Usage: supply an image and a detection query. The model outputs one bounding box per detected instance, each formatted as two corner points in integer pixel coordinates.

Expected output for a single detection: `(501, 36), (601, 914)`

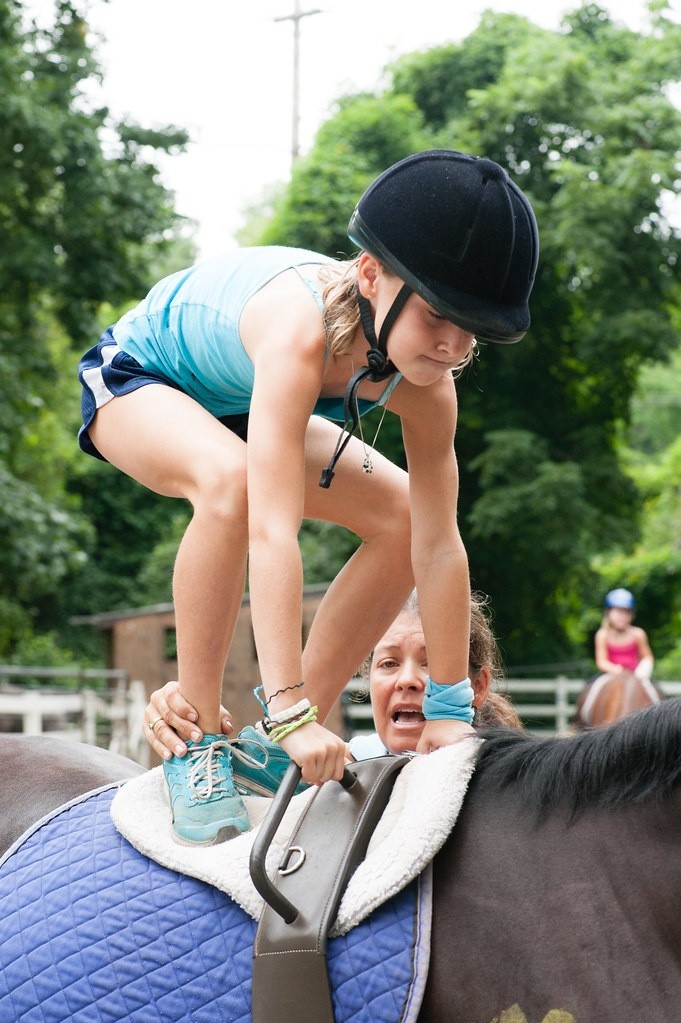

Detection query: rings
(148, 716), (163, 731)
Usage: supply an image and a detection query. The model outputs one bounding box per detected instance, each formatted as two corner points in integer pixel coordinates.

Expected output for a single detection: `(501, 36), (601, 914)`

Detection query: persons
(74, 149), (542, 849)
(141, 579), (531, 772)
(578, 588), (662, 725)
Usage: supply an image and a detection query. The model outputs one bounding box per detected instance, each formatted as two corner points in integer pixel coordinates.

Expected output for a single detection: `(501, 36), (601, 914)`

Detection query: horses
(0, 669), (681, 1023)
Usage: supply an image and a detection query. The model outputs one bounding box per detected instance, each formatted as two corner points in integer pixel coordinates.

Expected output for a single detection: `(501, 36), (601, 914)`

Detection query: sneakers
(231, 725), (316, 797)
(162, 734), (271, 848)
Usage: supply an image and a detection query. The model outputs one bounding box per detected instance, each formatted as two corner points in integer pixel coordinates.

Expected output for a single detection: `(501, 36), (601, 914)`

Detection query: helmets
(347, 148), (540, 344)
(604, 588), (635, 610)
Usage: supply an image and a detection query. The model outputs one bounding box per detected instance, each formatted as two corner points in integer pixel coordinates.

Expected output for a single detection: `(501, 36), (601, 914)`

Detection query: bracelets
(252, 679), (317, 743)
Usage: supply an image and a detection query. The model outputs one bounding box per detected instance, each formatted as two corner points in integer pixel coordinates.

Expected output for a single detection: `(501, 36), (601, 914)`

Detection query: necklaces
(350, 355), (396, 473)
